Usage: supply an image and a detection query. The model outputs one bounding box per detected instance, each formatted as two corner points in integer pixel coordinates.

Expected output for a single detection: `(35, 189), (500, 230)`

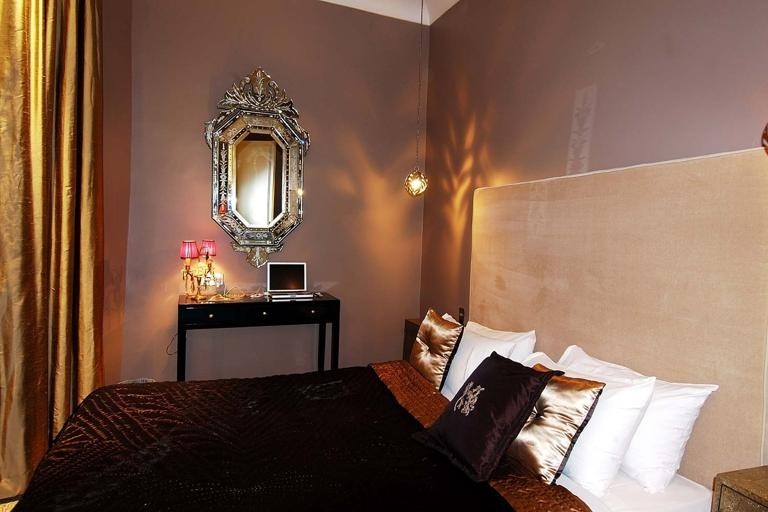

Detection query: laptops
(267, 262), (313, 299)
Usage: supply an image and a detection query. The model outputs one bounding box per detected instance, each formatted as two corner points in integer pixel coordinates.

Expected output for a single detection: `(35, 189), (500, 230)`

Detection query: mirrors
(204, 66), (310, 269)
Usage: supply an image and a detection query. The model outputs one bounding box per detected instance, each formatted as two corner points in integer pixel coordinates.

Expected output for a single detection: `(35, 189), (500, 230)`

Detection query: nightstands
(710, 465), (768, 511)
(404, 318), (423, 360)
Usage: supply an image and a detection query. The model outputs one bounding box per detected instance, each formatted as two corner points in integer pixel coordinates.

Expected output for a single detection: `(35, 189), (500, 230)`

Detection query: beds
(11, 146), (768, 512)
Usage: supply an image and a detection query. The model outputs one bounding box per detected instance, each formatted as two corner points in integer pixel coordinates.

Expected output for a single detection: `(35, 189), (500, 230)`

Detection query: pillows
(410, 309), (464, 393)
(557, 345), (719, 496)
(524, 353), (656, 499)
(508, 363), (606, 485)
(441, 312), (517, 402)
(466, 321), (537, 362)
(413, 351), (566, 486)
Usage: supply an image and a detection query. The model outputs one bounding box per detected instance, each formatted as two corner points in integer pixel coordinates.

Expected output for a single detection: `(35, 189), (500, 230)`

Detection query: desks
(178, 292), (340, 381)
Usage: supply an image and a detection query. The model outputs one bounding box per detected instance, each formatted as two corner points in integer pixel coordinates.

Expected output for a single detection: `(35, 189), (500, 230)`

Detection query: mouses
(315, 292), (324, 298)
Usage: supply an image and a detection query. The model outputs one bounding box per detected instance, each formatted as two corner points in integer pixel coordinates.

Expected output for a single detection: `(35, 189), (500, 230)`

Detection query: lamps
(179, 239), (217, 301)
(406, 0), (430, 198)
